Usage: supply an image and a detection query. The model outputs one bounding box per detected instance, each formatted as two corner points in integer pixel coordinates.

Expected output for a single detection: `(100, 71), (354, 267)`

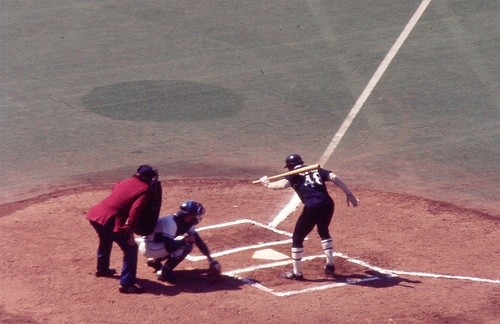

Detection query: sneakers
(323, 265), (335, 274)
(284, 272), (304, 280)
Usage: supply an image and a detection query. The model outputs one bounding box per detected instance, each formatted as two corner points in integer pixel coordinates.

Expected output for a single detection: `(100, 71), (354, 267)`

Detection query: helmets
(283, 154), (303, 169)
(137, 165), (158, 182)
(175, 200), (202, 218)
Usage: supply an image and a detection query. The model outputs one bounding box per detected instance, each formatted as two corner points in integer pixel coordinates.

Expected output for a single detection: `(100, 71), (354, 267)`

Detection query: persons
(259, 154), (360, 281)
(84, 164), (162, 293)
(140, 201), (220, 278)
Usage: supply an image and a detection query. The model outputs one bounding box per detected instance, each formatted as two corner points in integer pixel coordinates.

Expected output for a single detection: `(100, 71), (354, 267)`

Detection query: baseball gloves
(207, 259), (221, 283)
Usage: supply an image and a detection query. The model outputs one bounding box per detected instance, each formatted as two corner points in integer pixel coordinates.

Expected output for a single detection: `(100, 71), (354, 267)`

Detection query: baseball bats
(252, 163), (321, 184)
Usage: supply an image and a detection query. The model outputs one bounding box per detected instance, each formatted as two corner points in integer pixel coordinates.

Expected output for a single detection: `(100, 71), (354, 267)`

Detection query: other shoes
(157, 270), (181, 282)
(147, 259), (162, 272)
(96, 268), (116, 276)
(119, 283), (144, 293)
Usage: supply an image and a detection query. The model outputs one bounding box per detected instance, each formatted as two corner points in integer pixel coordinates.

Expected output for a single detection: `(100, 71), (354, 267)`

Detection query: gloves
(260, 176), (270, 187)
(347, 194), (358, 207)
